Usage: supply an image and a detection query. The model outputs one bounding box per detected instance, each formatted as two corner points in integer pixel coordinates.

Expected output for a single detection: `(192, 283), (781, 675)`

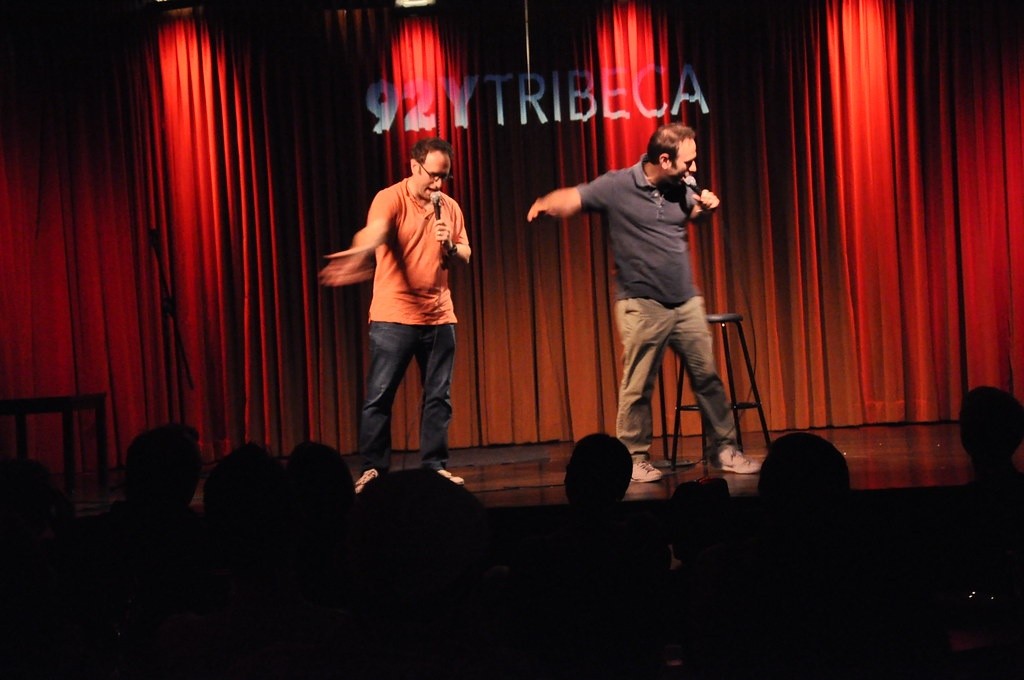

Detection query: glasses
(416, 159), (454, 185)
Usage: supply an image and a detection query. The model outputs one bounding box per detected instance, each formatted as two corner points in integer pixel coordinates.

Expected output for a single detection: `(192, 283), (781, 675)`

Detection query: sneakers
(629, 461), (664, 483)
(710, 444), (767, 474)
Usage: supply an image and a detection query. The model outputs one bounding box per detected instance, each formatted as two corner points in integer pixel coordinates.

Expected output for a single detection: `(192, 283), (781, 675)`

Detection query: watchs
(447, 245), (457, 255)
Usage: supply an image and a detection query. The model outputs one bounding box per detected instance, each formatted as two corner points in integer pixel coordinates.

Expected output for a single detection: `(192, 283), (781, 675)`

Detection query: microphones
(684, 175), (702, 196)
(430, 192), (442, 221)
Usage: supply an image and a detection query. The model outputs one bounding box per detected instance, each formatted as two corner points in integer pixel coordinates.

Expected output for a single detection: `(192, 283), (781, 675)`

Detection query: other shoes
(437, 469), (464, 485)
(355, 469), (378, 493)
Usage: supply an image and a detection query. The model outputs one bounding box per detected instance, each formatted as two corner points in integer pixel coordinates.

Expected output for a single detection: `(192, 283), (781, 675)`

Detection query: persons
(527, 124), (762, 482)
(320, 138), (472, 491)
(0, 385), (1024, 680)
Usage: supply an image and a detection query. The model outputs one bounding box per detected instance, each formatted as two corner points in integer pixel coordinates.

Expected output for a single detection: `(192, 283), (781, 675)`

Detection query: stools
(668, 314), (771, 469)
